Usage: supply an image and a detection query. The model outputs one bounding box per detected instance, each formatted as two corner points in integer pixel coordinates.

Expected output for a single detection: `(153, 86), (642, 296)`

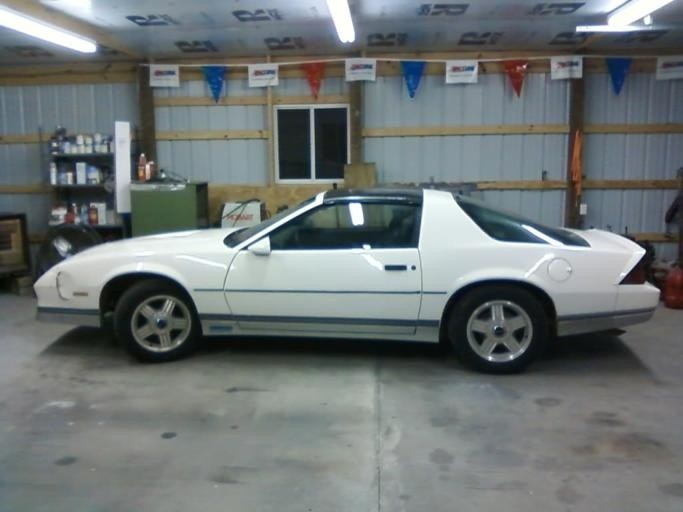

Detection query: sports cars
(32, 185), (661, 374)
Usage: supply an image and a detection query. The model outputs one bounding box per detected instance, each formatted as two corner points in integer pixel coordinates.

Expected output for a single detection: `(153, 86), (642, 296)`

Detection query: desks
(130, 180), (210, 239)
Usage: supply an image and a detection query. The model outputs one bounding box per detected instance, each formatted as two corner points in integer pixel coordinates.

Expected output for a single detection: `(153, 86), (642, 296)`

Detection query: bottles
(138, 153), (147, 179)
(48, 160), (74, 185)
(66, 202), (99, 225)
(50, 125), (110, 154)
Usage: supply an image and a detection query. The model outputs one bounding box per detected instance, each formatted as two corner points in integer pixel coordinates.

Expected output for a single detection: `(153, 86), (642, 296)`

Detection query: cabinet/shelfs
(48, 136), (130, 264)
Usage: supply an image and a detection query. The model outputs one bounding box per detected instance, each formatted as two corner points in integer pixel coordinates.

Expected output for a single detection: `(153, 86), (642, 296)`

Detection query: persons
(665, 167), (683, 268)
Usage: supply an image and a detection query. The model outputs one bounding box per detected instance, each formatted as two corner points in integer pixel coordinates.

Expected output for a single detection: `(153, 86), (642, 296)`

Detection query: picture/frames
(1, 212), (34, 280)
